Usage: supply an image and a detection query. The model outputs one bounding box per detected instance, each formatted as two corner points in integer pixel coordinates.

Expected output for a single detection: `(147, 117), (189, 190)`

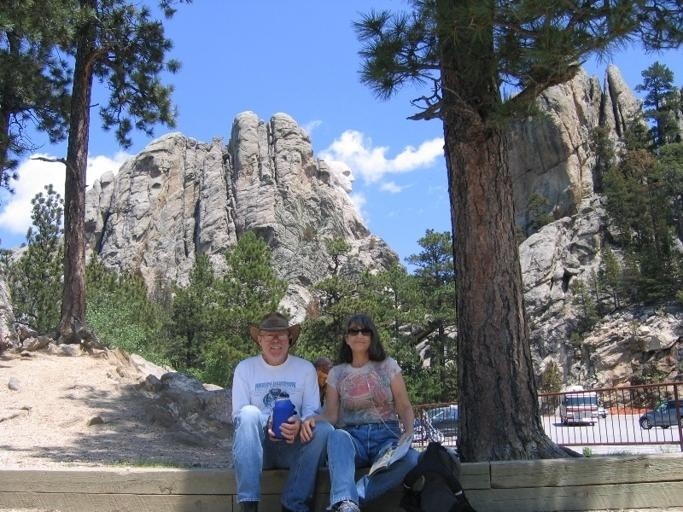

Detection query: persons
(299, 313), (422, 512)
(229, 311), (336, 512)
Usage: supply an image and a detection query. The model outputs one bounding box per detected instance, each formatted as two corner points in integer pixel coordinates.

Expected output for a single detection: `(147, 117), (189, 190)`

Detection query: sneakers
(338, 499), (362, 511)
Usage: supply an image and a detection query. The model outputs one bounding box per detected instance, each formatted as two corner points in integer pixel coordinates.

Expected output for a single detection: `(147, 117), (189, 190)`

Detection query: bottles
(272, 392), (294, 440)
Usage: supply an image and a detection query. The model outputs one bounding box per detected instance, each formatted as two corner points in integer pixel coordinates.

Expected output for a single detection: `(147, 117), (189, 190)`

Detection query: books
(368, 434), (413, 477)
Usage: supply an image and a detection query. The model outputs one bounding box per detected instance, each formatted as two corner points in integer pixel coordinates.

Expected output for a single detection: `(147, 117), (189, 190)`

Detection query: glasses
(257, 331), (289, 342)
(347, 327), (373, 337)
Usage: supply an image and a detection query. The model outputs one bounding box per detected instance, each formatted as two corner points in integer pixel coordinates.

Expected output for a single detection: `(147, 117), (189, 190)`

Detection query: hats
(247, 312), (301, 348)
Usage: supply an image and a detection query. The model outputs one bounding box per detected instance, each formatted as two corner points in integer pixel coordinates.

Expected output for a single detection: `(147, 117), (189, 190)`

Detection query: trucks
(556, 385), (598, 427)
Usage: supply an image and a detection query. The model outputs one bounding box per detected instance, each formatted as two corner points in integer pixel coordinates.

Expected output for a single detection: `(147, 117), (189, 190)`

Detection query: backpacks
(402, 442), (472, 512)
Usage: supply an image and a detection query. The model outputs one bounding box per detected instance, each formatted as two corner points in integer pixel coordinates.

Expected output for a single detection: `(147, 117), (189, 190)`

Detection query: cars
(638, 399), (683, 430)
(597, 406), (608, 419)
(414, 404), (458, 435)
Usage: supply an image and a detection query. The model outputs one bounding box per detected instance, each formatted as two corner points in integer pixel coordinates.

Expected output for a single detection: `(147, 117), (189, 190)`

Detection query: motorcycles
(398, 409), (444, 445)
